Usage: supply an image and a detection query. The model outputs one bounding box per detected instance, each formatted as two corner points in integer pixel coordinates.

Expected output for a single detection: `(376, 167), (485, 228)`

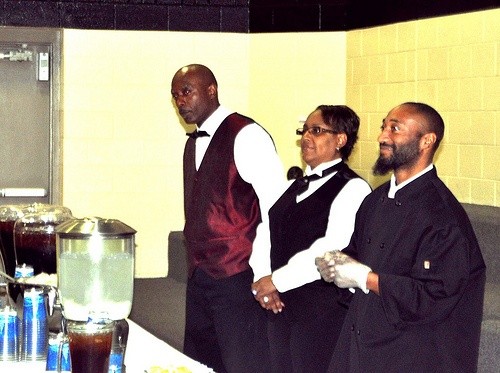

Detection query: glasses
(296, 127), (337, 137)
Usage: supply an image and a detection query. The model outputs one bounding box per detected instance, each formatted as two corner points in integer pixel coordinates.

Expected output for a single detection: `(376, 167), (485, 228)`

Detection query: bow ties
(186, 130), (210, 139)
(286, 166), (310, 195)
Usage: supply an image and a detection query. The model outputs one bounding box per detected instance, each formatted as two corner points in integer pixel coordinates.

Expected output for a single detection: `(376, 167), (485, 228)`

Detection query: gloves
(330, 249), (372, 295)
(314, 250), (335, 283)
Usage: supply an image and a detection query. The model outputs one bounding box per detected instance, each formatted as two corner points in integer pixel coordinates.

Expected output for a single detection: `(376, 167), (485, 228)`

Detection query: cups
(0, 267), (124, 373)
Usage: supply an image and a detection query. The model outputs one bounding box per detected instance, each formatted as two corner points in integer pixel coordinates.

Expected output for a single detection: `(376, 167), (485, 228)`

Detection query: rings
(263, 296), (269, 302)
(252, 289), (257, 296)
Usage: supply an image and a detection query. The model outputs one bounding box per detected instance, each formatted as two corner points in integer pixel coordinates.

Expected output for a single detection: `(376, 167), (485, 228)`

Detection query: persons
(171, 64), (291, 373)
(315, 101), (486, 373)
(249, 105), (373, 373)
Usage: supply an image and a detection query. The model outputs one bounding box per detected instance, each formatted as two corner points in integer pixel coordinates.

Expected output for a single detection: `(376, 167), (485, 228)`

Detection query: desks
(0, 318), (216, 373)
